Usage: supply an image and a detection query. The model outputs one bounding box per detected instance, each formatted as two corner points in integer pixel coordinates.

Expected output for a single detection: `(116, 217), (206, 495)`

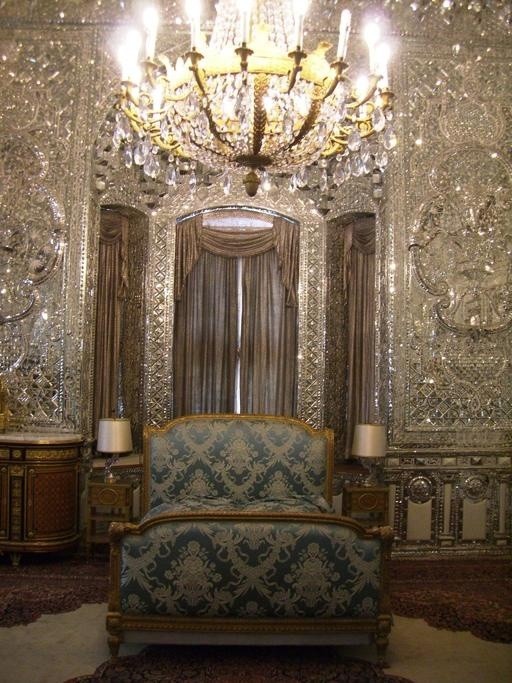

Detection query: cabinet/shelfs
(0, 432), (85, 567)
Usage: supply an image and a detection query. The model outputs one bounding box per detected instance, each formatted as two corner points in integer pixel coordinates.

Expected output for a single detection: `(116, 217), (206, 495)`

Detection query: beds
(108, 414), (394, 669)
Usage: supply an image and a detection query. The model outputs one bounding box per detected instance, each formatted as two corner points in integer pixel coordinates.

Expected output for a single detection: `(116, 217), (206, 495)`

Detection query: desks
(343, 483), (390, 560)
(86, 477), (142, 563)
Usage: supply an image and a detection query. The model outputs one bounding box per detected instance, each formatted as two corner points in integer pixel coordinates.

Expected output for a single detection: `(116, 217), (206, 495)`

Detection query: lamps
(97, 418), (133, 483)
(112, 0), (398, 196)
(351, 422), (387, 488)
